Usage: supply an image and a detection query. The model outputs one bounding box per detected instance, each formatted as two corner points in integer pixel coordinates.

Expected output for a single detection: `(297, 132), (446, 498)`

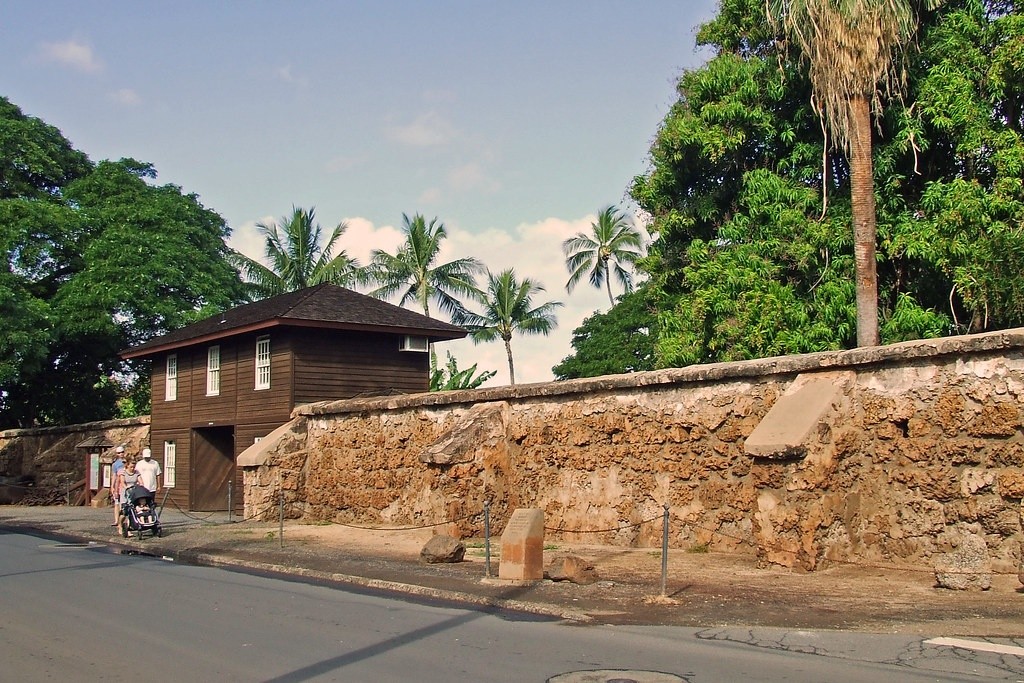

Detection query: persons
(109, 446), (129, 527)
(117, 459), (144, 537)
(135, 497), (155, 526)
(134, 448), (162, 507)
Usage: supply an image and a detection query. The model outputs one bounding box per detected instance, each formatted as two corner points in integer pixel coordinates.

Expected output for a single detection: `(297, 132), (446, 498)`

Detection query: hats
(143, 449), (152, 457)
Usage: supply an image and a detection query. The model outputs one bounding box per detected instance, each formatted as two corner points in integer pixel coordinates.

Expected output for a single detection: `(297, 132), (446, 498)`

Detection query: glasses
(117, 451), (124, 454)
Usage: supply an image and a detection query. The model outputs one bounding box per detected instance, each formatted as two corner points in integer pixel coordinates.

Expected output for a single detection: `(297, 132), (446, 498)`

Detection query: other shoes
(111, 522), (118, 526)
(128, 534), (133, 536)
(119, 528), (122, 535)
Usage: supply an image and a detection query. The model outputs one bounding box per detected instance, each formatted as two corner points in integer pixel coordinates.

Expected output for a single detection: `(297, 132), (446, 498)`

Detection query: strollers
(120, 485), (163, 540)
